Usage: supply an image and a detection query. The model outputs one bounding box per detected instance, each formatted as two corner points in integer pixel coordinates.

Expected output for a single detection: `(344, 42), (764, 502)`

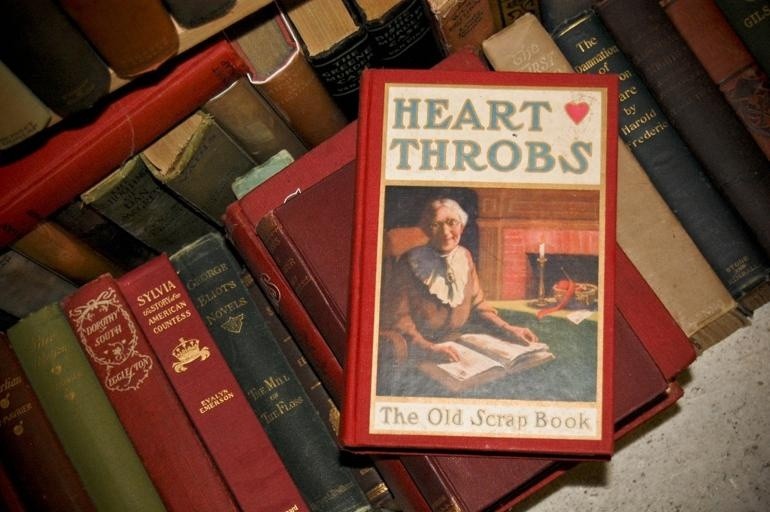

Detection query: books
(0, 0), (769, 512)
